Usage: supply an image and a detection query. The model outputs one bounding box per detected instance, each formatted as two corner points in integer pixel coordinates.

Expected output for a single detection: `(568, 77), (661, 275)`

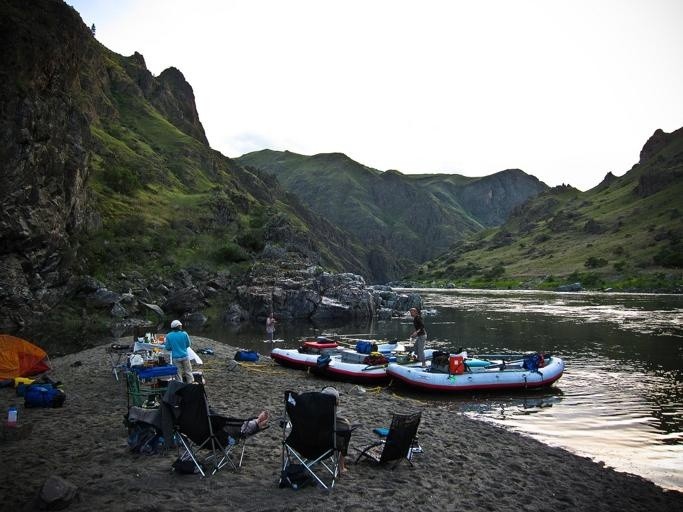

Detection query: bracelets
(416, 330), (417, 333)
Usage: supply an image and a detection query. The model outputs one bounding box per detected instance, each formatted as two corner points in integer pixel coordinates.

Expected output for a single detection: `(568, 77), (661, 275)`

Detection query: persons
(409, 307), (426, 366)
(163, 320), (194, 384)
(265, 312), (275, 340)
(321, 386), (350, 472)
(209, 406), (270, 446)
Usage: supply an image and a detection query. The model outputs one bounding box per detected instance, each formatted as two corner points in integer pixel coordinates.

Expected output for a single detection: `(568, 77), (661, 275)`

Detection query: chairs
(124, 370), (422, 492)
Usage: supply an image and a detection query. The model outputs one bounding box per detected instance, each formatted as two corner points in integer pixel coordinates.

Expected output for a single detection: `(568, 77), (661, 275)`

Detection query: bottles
(7, 407), (17, 428)
(142, 350), (165, 369)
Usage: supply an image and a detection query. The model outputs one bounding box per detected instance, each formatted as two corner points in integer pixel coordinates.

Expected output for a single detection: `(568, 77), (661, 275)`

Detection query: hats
(170, 319), (182, 329)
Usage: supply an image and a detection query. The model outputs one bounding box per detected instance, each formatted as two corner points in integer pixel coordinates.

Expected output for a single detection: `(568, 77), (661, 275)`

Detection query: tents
(0, 334), (53, 390)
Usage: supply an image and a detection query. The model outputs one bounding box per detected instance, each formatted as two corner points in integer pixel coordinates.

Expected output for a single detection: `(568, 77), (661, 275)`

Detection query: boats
(270, 336), (564, 398)
